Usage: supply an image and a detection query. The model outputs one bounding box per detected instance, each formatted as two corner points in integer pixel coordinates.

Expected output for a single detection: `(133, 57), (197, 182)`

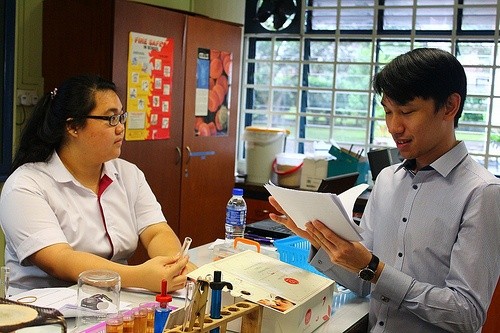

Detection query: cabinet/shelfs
(233, 179), (369, 226)
(42, 0), (247, 266)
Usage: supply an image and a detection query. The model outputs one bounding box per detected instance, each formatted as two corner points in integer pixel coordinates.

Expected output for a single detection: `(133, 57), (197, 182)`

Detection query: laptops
(244, 172), (360, 239)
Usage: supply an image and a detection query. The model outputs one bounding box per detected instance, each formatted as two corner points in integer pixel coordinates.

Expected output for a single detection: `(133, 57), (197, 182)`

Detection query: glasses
(87, 110), (129, 128)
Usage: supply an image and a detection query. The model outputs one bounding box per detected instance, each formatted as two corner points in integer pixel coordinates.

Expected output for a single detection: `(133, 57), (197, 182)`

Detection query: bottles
(224, 188), (247, 249)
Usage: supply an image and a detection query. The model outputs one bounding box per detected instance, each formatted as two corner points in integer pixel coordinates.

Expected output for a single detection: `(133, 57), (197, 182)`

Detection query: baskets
(271, 236), (353, 296)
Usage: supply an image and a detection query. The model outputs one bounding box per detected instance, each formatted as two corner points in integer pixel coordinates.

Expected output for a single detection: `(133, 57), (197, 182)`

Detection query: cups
(73, 270), (121, 333)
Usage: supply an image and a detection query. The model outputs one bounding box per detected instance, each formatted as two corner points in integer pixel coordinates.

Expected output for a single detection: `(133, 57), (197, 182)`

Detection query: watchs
(357, 254), (380, 282)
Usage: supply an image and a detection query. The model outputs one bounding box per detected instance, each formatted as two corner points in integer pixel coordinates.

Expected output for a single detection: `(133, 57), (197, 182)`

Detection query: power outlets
(17, 89), (38, 109)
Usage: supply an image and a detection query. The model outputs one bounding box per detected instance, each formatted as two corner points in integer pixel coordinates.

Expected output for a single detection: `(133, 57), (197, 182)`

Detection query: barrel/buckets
(272, 153), (306, 188)
(241, 125), (291, 186)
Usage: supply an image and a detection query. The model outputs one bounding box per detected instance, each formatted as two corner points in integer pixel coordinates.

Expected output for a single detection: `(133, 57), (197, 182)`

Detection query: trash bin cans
(243, 126), (291, 184)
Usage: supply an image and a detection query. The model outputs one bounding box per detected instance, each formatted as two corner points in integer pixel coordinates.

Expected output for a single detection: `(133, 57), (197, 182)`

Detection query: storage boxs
(300, 157), (356, 192)
(187, 250), (336, 332)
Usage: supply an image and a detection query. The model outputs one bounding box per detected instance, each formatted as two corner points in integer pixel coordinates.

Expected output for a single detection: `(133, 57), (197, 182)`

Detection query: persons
(259, 295), (295, 313)
(0, 76), (197, 299)
(268, 48), (500, 333)
